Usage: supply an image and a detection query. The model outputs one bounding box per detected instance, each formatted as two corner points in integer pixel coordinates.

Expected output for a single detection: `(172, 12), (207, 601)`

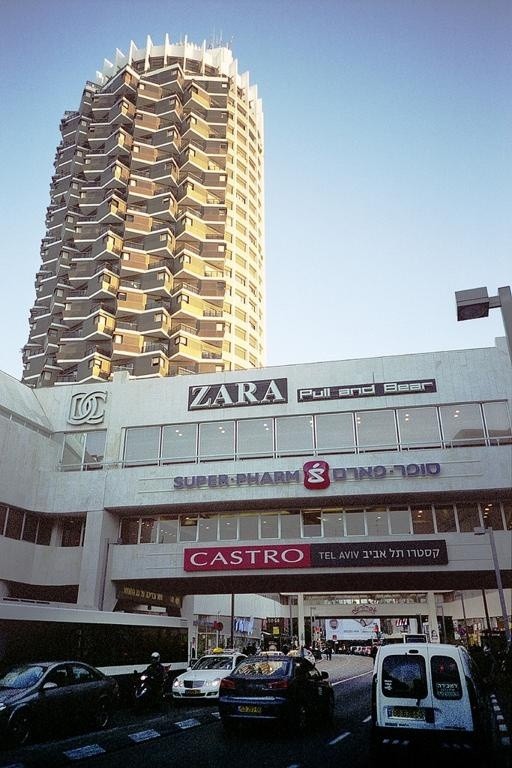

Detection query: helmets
(150, 652), (160, 662)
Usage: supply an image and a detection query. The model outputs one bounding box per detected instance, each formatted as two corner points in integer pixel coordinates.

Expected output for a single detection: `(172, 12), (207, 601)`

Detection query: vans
(369, 633), (487, 731)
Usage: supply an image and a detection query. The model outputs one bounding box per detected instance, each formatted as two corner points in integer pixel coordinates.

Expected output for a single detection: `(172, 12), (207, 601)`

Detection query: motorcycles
(128, 664), (173, 712)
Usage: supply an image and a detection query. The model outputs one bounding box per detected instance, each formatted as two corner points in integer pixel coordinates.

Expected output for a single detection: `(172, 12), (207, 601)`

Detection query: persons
(139, 650), (166, 697)
(326, 645), (333, 661)
(217, 636), (298, 656)
(371, 644), (378, 666)
(467, 640), (492, 670)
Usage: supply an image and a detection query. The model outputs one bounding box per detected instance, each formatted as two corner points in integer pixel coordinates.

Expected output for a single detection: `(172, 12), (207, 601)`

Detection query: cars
(349, 646), (378, 656)
(0, 661), (120, 745)
(172, 647), (335, 727)
